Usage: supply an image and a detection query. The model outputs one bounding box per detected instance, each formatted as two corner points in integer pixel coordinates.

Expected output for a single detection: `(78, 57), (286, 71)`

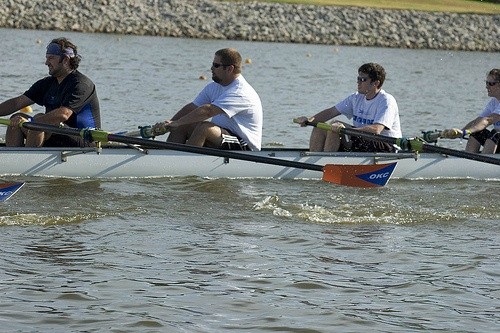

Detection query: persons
(152, 48), (263, 152)
(461, 68), (500, 154)
(297, 63), (403, 152)
(0, 37), (101, 148)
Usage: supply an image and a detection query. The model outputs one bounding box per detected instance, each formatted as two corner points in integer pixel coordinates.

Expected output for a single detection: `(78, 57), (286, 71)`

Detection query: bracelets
(463, 129), (471, 136)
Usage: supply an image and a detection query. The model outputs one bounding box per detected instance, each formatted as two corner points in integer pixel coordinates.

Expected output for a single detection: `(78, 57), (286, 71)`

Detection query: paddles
(423, 130), (471, 143)
(115, 122), (169, 137)
(293, 118), (500, 166)
(1, 178), (25, 205)
(1, 119), (399, 187)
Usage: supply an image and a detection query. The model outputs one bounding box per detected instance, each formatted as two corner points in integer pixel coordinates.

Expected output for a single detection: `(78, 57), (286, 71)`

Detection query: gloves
(443, 128), (461, 139)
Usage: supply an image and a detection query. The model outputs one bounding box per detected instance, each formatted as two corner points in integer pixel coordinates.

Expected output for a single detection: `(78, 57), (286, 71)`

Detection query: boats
(0, 106), (500, 180)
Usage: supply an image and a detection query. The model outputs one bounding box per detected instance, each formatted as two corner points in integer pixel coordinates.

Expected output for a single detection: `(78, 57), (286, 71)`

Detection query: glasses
(212, 62), (228, 68)
(486, 81), (500, 86)
(357, 76), (372, 82)
